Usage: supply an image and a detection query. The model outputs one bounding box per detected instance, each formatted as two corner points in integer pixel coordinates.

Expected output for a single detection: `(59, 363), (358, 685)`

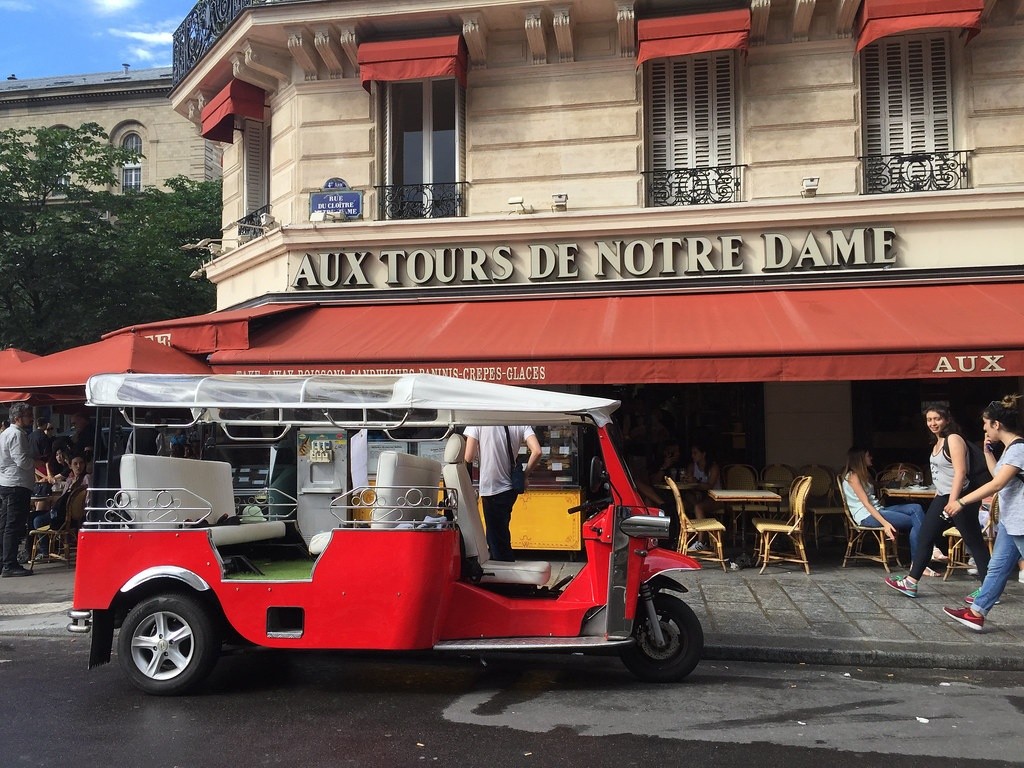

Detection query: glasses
(24, 414), (33, 417)
(46, 427), (54, 431)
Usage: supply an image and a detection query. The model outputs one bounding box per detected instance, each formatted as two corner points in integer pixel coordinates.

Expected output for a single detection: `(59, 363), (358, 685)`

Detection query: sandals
(931, 548), (950, 563)
(910, 564), (941, 577)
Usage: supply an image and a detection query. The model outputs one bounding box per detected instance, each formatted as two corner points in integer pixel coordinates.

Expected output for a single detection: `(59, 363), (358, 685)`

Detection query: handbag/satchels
(50, 489), (72, 530)
(511, 466), (525, 494)
(688, 490), (707, 505)
(943, 433), (994, 487)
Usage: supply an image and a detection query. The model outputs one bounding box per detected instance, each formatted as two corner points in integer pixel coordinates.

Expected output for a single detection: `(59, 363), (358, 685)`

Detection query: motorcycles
(68, 368), (706, 695)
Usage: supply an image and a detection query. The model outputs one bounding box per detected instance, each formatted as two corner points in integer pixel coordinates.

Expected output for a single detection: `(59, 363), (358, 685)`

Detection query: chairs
(27, 482), (89, 573)
(443, 433), (553, 586)
(661, 460), (1000, 582)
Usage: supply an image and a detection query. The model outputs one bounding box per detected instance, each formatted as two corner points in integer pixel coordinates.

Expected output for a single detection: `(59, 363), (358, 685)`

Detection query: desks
(707, 488), (782, 570)
(879, 484), (938, 566)
(650, 481), (700, 549)
(28, 495), (63, 563)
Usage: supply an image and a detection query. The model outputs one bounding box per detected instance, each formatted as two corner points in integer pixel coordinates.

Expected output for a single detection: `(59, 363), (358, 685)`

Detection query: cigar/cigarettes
(886, 538), (894, 540)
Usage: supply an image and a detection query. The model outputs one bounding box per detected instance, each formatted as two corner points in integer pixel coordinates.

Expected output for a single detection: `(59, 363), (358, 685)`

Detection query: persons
(942, 392), (1024, 632)
(886, 403), (1001, 603)
(463, 426), (540, 562)
(842, 444), (1024, 583)
(0, 401), (194, 577)
(624, 444), (721, 551)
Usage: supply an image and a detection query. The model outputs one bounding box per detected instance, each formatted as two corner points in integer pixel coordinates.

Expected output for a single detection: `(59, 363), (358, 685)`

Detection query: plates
(908, 486), (929, 490)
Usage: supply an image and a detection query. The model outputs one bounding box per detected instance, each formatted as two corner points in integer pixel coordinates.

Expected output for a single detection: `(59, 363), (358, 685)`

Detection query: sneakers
(687, 541), (711, 551)
(964, 586), (1000, 604)
(884, 576), (918, 597)
(943, 607), (984, 631)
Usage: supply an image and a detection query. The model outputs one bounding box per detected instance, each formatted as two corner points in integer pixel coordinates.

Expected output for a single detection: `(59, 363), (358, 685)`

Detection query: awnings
(851, 0), (985, 52)
(200, 79), (265, 143)
(635, 6), (752, 72)
(100, 278), (1024, 384)
(357, 33), (468, 94)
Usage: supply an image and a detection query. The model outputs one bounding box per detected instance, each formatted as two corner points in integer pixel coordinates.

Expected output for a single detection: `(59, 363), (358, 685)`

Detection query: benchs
(118, 453), (285, 546)
(307, 450), (441, 554)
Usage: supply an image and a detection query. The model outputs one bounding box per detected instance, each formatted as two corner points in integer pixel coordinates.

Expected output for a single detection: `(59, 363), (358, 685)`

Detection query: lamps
(180, 213), (281, 280)
(310, 211), (344, 222)
(508, 197), (535, 215)
(800, 177), (820, 198)
(551, 193), (569, 212)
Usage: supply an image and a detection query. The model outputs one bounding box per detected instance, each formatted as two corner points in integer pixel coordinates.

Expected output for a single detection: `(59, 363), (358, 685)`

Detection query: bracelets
(958, 499), (966, 507)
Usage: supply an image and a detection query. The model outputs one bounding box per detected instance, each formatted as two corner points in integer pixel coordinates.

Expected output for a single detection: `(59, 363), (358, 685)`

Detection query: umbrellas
(0, 328), (217, 509)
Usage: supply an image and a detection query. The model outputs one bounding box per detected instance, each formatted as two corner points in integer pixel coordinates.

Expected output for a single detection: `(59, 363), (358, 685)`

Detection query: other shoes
(1019, 570), (1024, 584)
(0, 562), (32, 576)
(31, 553), (48, 563)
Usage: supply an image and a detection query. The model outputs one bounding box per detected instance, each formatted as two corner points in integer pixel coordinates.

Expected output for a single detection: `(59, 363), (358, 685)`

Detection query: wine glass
(913, 471), (923, 489)
